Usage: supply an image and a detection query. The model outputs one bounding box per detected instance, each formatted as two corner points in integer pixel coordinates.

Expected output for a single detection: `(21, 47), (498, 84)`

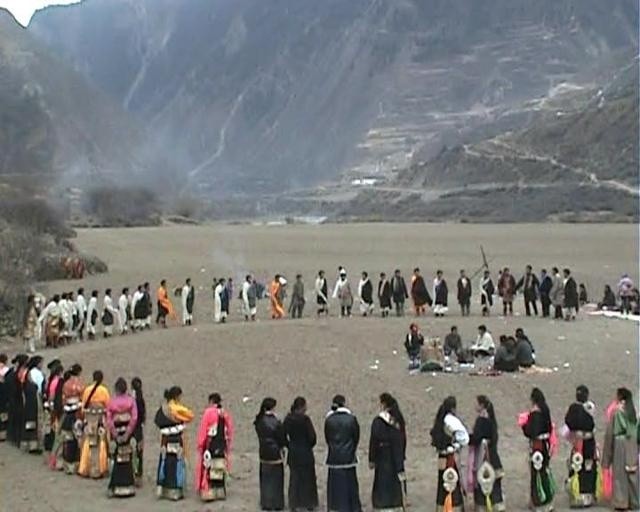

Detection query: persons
(0, 353), (148, 498)
(153, 386), (195, 501)
(23, 263), (579, 353)
(195, 394), (233, 501)
(577, 274), (640, 316)
(430, 394), (506, 512)
(404, 323), (535, 376)
(254, 392), (407, 512)
(518, 385), (640, 512)
